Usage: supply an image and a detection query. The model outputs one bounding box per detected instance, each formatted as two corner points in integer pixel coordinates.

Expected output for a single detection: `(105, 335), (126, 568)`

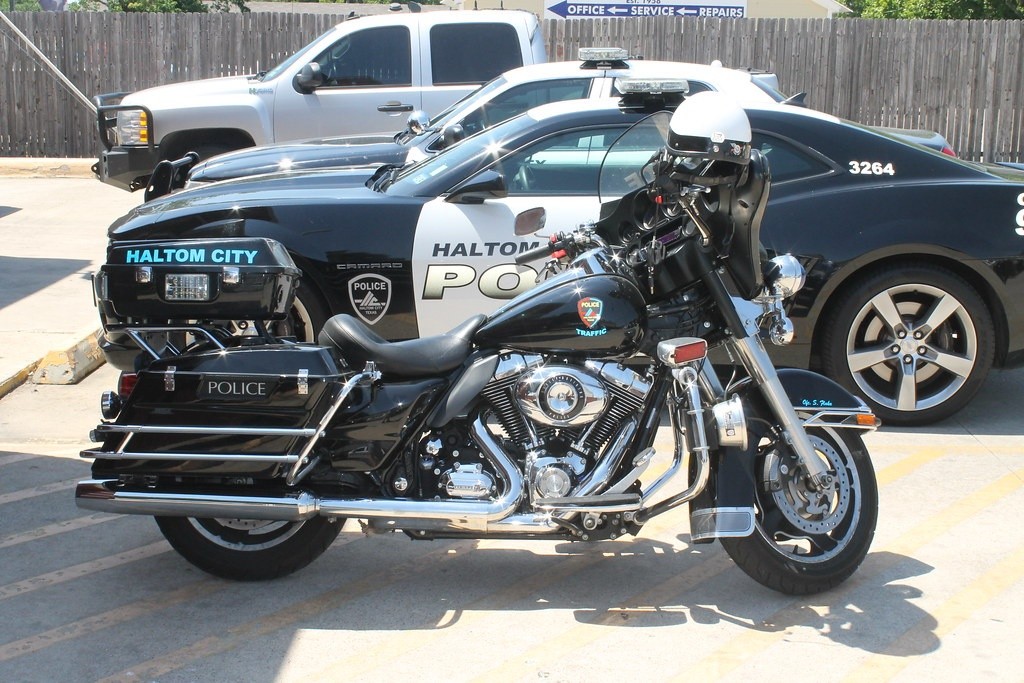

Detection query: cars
(91, 76), (1024, 428)
(141, 46), (962, 206)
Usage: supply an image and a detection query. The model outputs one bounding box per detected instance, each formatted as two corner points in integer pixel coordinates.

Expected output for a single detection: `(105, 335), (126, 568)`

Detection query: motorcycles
(75, 101), (883, 599)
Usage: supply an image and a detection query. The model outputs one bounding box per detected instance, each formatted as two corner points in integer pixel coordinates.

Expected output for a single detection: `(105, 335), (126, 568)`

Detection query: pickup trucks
(89, 9), (781, 193)
